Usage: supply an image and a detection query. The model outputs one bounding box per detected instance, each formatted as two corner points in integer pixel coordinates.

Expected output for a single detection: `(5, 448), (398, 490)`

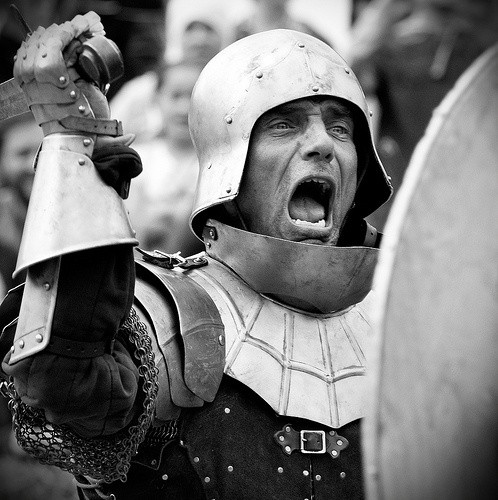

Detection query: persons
(108, 18), (226, 123)
(1, 118), (48, 295)
(231, 0), (332, 43)
(121, 61), (201, 258)
(0, 8), (394, 500)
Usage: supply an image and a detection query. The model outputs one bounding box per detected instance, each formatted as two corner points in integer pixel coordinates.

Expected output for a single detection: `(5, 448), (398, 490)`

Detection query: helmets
(188, 29), (392, 244)
